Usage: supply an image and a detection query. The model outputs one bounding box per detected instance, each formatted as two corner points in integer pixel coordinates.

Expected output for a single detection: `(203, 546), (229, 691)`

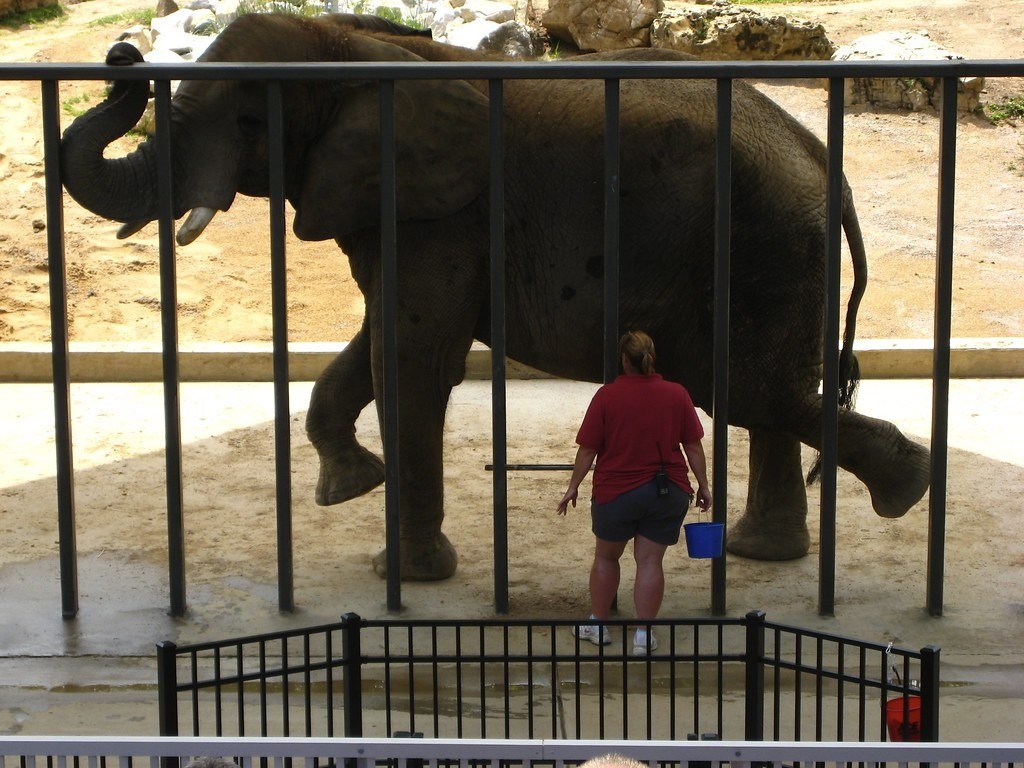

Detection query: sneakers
(572, 615), (612, 645)
(634, 627), (658, 656)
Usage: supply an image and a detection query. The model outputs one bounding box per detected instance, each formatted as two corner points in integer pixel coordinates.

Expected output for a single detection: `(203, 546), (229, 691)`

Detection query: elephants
(57, 11), (928, 583)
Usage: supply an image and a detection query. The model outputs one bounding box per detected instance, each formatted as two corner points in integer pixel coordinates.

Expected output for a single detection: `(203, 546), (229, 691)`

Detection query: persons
(557, 332), (713, 657)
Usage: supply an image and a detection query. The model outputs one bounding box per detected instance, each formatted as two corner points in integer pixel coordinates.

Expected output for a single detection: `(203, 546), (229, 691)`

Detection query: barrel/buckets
(683, 522), (725, 559)
(887, 696), (921, 742)
